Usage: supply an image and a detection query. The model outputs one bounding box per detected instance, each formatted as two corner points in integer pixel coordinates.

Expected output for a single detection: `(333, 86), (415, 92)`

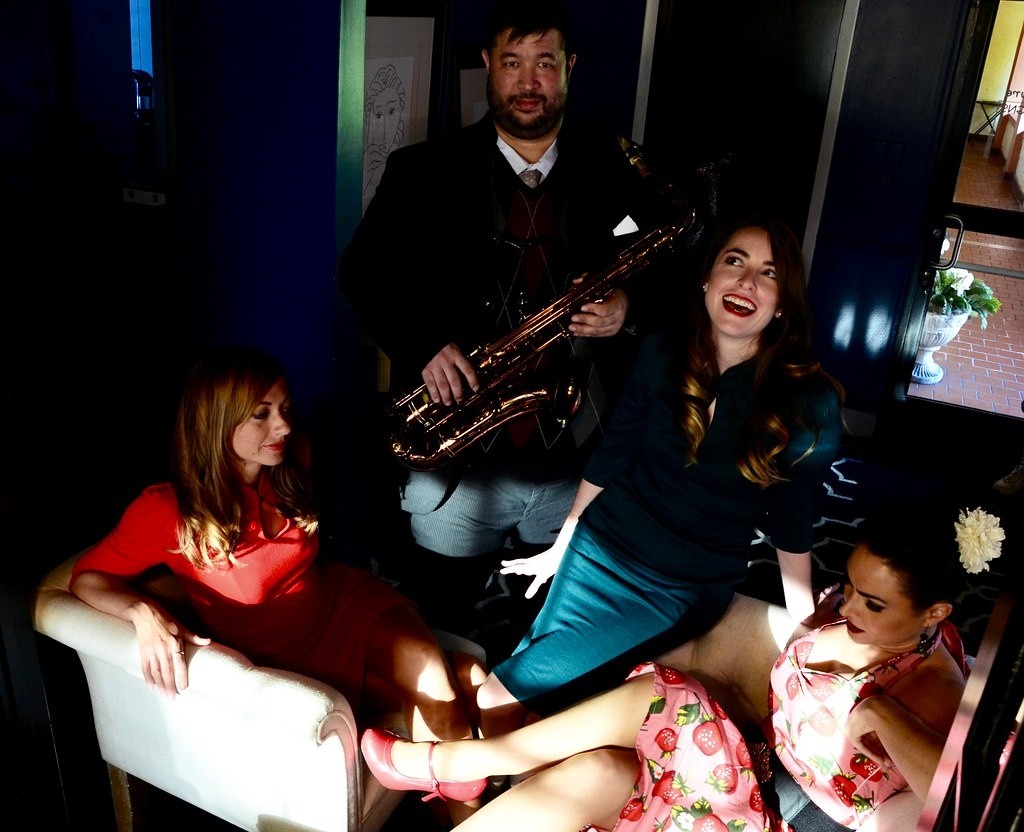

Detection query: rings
(174, 649), (184, 655)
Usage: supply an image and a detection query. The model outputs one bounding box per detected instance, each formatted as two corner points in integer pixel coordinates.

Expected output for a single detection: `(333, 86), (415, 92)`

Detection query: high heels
(360, 728), (489, 802)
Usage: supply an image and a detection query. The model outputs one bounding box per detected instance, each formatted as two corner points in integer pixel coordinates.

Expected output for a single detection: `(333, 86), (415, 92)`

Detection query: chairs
(32, 538), (486, 832)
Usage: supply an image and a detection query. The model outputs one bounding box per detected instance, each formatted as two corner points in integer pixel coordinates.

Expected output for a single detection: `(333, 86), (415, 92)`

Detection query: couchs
(648, 593), (924, 832)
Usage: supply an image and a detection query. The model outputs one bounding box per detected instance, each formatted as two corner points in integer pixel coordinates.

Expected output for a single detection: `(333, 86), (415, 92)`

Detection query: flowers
(953, 506), (1006, 575)
(928, 239), (1002, 330)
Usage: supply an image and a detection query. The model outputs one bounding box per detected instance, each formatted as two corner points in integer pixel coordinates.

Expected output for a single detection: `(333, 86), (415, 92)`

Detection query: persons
(69, 7), (968, 832)
(361, 490), (1005, 832)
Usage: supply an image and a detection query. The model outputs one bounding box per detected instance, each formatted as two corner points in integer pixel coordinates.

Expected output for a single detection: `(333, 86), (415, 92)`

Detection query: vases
(912, 306), (970, 384)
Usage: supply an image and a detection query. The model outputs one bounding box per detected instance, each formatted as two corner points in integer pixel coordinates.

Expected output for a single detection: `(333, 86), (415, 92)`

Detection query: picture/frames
(361, 0), (450, 219)
(452, 59), (490, 129)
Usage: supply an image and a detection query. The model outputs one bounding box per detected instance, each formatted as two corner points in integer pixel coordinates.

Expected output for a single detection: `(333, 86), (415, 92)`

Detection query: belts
(747, 727), (852, 832)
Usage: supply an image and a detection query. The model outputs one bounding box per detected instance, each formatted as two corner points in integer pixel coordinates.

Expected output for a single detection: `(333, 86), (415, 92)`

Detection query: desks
(968, 100), (1003, 143)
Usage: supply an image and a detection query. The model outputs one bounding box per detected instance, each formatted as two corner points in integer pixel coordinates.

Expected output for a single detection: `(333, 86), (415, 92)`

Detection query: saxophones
(383, 133), (707, 471)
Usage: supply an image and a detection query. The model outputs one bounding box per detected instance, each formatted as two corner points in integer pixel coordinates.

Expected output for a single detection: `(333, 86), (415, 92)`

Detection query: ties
(519, 170), (542, 190)
